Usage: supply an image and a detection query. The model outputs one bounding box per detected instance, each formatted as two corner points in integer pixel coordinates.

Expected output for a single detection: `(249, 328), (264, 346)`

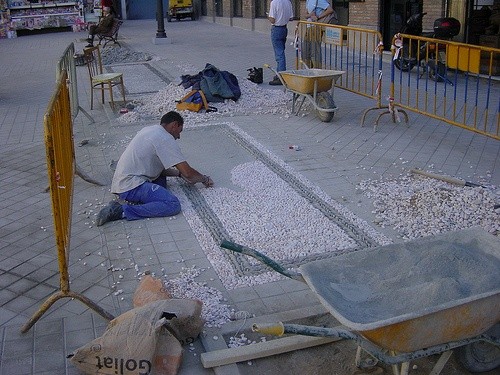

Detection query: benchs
(97, 18), (124, 49)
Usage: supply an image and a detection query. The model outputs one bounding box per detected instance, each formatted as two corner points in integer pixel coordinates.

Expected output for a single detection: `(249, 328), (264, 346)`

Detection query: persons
(84, 7), (114, 48)
(269, 0), (293, 85)
(303, 0), (334, 69)
(97, 111), (214, 226)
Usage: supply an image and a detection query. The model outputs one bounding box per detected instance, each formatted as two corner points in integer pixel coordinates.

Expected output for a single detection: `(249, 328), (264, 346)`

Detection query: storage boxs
(433, 18), (460, 38)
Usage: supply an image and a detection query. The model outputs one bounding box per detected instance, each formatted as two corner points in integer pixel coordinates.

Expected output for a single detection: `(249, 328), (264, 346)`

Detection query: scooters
(394, 11), (461, 82)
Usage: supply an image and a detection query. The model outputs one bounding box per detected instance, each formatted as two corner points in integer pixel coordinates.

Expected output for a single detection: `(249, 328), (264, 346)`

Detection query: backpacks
(175, 89), (209, 113)
(314, 0), (339, 32)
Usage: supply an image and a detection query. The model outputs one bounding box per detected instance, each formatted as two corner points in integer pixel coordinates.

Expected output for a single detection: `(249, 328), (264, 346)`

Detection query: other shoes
(269, 75), (283, 85)
(85, 44), (94, 47)
(85, 38), (93, 43)
(96, 201), (124, 227)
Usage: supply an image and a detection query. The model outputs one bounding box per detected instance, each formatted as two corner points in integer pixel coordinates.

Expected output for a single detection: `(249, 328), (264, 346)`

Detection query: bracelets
(178, 170), (182, 178)
(202, 175), (210, 185)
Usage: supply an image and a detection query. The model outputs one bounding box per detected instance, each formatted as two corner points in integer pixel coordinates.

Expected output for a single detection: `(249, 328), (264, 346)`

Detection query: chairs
(82, 43), (125, 113)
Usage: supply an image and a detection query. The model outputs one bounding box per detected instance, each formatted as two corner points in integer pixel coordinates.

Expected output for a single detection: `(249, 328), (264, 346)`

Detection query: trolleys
(263, 59), (347, 123)
(218, 225), (500, 375)
